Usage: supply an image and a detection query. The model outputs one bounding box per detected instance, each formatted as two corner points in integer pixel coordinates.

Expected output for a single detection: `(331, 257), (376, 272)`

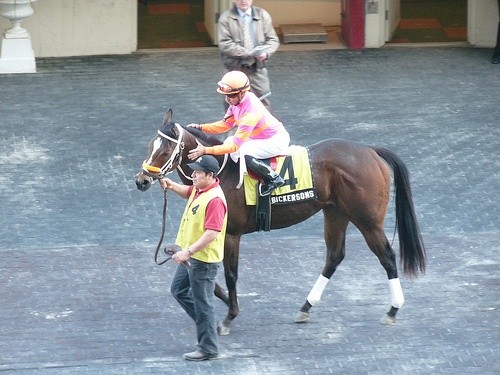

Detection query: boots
(249, 158), (286, 197)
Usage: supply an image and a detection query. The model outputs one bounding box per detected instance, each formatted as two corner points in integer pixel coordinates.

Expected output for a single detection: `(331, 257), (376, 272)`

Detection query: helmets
(216, 71), (251, 95)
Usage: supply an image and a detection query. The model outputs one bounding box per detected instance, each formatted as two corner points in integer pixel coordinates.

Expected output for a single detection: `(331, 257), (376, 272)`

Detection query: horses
(135, 108), (427, 336)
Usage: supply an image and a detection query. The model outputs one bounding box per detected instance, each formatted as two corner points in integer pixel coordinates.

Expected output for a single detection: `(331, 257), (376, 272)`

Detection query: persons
(217, 0), (279, 115)
(159, 155), (228, 360)
(187, 70), (290, 196)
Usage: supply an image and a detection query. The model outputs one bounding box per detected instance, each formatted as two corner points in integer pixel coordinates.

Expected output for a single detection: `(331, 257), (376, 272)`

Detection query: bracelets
(187, 248), (193, 256)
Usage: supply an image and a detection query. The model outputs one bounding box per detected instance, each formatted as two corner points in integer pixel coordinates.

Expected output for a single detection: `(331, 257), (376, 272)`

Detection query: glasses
(226, 94), (237, 98)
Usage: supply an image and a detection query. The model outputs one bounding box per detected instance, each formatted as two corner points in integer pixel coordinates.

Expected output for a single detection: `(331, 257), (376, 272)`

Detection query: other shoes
(183, 350), (218, 361)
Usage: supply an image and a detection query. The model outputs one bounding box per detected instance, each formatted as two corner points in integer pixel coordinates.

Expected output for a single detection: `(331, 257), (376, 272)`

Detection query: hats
(185, 154), (220, 172)
